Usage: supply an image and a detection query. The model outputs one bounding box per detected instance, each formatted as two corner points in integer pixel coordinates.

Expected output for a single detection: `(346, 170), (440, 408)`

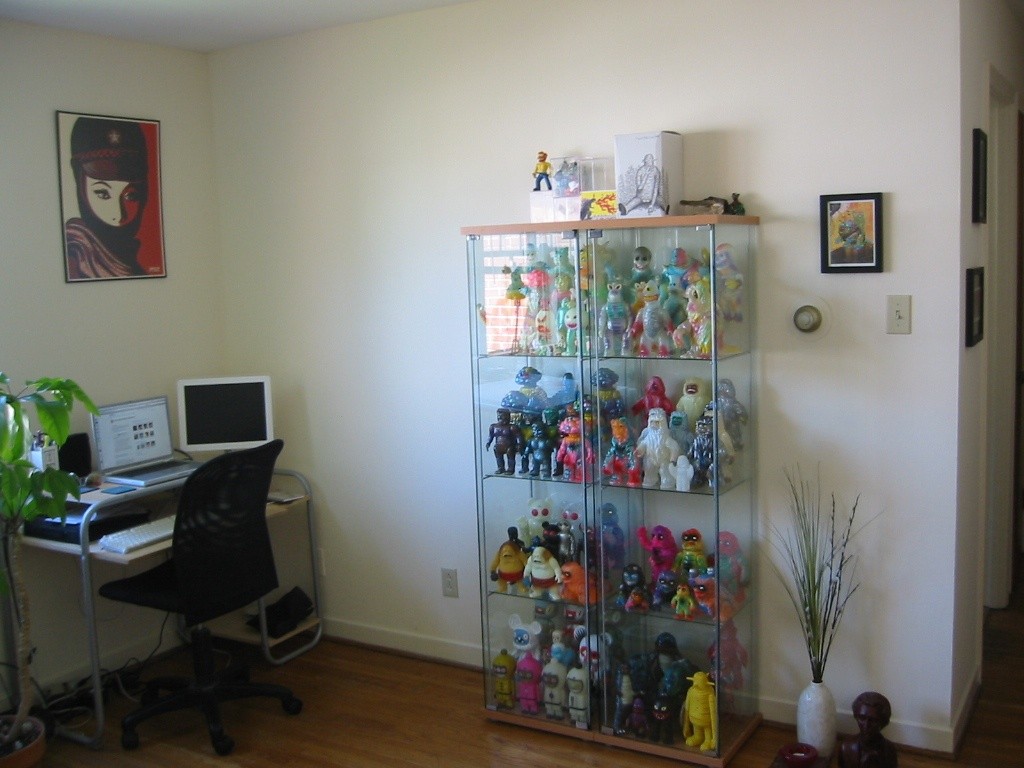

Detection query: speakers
(59, 433), (92, 476)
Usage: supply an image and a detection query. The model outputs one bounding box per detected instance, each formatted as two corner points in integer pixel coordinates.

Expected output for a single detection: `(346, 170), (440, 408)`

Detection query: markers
(30, 430), (56, 451)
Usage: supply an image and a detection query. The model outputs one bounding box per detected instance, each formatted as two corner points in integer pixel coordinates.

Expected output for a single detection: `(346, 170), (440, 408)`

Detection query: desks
(13, 467), (325, 744)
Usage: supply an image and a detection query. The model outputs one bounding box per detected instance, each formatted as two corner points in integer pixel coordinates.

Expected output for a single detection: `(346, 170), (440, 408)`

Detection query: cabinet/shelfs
(462, 215), (763, 768)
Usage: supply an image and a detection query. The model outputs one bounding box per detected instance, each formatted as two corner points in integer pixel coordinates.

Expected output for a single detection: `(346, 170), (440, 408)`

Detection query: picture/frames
(55, 111), (168, 284)
(966, 266), (984, 347)
(973, 128), (987, 224)
(821, 192), (884, 274)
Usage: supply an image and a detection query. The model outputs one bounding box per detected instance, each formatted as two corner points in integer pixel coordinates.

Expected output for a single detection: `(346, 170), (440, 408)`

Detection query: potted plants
(0, 372), (100, 768)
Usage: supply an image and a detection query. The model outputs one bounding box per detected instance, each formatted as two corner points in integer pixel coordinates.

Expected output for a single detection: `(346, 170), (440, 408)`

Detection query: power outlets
(441, 568), (459, 597)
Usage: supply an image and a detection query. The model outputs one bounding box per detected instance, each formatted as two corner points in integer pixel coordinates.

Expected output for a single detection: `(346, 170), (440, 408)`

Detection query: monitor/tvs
(177, 376), (274, 451)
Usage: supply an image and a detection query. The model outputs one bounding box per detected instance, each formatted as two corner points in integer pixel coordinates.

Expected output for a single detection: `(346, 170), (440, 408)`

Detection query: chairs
(99, 438), (304, 756)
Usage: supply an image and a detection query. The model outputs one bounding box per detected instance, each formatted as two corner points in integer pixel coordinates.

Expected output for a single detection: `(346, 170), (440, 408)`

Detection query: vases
(797, 681), (836, 758)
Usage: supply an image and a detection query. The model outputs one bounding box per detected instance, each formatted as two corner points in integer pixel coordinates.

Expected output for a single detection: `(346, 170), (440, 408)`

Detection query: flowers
(759, 459), (888, 684)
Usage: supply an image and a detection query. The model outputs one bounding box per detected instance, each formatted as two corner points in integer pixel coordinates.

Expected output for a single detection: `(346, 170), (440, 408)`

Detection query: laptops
(90, 396), (205, 485)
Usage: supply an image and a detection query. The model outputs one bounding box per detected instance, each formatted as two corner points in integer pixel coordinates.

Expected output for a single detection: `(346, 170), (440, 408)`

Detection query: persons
(533, 151), (551, 190)
(838, 692), (898, 768)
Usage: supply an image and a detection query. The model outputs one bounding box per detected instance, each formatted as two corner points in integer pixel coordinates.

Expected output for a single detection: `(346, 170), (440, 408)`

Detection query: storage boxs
(529, 131), (685, 224)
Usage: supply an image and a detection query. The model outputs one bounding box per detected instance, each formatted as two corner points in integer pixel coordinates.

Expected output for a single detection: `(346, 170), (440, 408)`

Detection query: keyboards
(99, 515), (177, 554)
